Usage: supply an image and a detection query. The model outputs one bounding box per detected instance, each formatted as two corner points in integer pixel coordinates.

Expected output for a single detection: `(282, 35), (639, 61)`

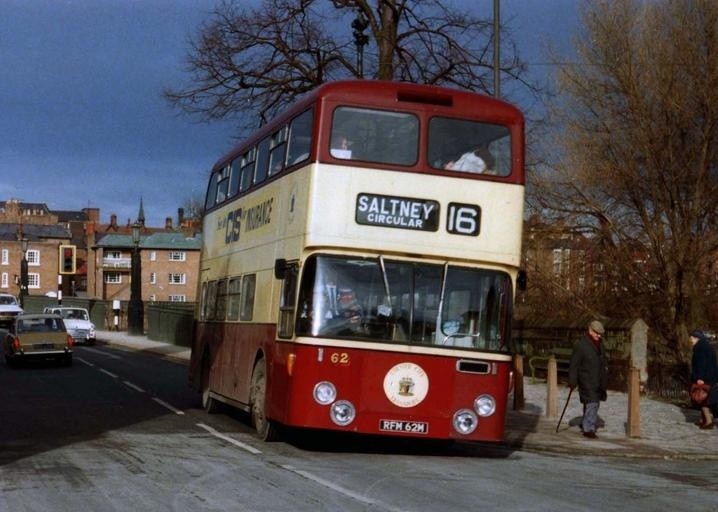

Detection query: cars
(0, 295), (23, 324)
(43, 307), (96, 346)
(0, 314), (75, 371)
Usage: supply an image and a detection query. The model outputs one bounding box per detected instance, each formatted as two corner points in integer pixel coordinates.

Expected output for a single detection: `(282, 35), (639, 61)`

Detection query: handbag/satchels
(691, 383), (712, 404)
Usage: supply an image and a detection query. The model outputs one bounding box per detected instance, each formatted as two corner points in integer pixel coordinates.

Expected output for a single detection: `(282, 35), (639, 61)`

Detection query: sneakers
(583, 431), (598, 439)
(695, 420), (713, 429)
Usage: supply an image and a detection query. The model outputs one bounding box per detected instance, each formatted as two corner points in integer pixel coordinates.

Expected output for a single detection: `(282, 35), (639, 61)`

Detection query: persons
(568, 321), (609, 438)
(689, 328), (717, 429)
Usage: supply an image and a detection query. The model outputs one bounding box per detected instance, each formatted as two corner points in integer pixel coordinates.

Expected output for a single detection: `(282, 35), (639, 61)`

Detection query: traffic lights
(57, 244), (77, 276)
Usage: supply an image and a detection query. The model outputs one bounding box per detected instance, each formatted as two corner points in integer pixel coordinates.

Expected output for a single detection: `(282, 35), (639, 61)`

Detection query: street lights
(127, 221), (143, 337)
(16, 234), (29, 306)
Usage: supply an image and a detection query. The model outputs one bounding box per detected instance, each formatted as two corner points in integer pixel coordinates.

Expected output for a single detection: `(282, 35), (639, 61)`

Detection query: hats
(591, 320), (605, 334)
(690, 329), (704, 338)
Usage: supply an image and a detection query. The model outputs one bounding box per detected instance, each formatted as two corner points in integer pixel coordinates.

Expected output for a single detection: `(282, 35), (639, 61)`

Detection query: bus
(186, 77), (527, 445)
